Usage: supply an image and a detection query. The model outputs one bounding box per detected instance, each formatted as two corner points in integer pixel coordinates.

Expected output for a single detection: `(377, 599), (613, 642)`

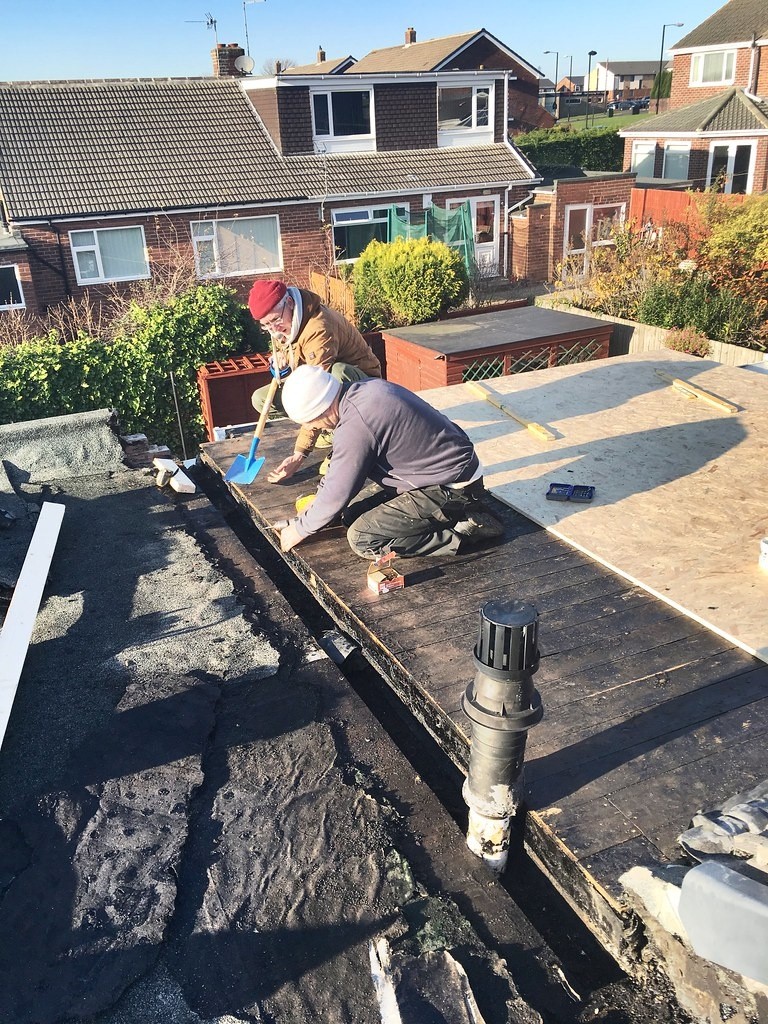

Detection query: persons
(247, 279), (383, 486)
(279, 363), (506, 560)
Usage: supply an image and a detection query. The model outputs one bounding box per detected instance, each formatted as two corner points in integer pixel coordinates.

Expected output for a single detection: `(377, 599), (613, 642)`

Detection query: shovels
(222, 365), (285, 486)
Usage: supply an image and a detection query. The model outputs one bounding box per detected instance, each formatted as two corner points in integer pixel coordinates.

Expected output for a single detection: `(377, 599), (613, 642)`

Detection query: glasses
(260, 301), (285, 330)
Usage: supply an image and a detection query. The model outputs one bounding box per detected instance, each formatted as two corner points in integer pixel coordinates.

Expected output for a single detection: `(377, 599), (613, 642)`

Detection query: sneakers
(454, 513), (504, 544)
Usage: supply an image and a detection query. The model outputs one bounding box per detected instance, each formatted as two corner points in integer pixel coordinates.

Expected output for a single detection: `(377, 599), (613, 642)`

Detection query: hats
(280, 364), (341, 425)
(249, 280), (286, 319)
